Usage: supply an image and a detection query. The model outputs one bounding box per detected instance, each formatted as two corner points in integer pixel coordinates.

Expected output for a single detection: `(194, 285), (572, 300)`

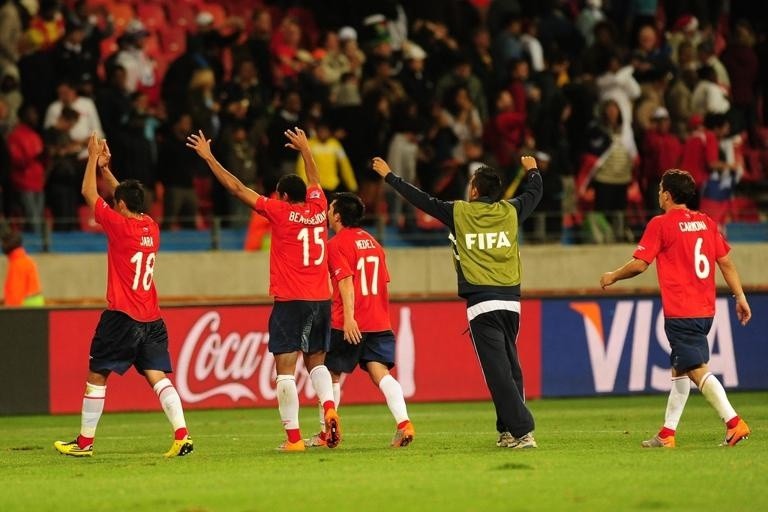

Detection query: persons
(0, 232), (46, 308)
(54, 132), (193, 457)
(186, 124), (339, 454)
(374, 157), (549, 451)
(0, 0), (768, 230)
(598, 168), (753, 449)
(304, 192), (413, 448)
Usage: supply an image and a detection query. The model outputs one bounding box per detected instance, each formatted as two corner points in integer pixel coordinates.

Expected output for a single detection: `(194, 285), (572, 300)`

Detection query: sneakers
(509, 433), (537, 450)
(392, 422), (414, 448)
(323, 409), (341, 449)
(721, 420), (751, 446)
(641, 433), (675, 449)
(273, 438), (305, 452)
(496, 432), (514, 447)
(54, 439), (93, 457)
(163, 436), (193, 457)
(305, 434), (328, 447)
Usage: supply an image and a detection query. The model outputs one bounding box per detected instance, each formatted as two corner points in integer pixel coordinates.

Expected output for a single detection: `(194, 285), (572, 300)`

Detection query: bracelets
(731, 292), (743, 298)
